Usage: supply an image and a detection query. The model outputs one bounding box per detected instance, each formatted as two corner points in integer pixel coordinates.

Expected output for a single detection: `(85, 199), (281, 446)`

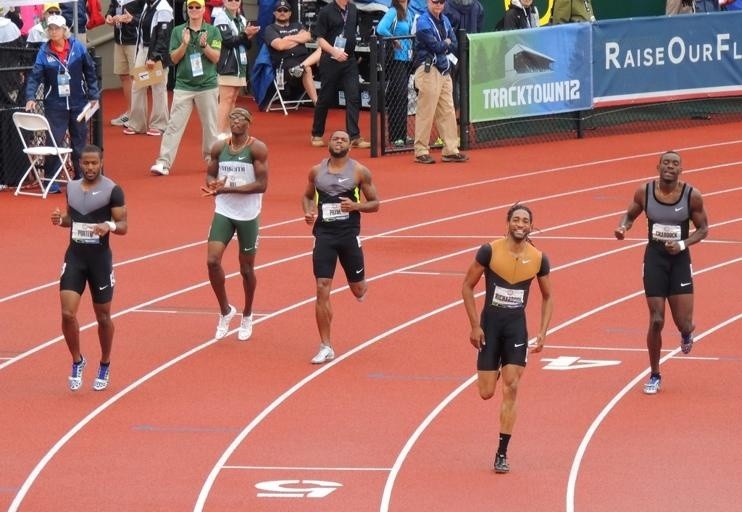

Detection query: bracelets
(106, 218), (118, 234)
(619, 223), (630, 233)
(678, 237), (689, 254)
(58, 216), (67, 226)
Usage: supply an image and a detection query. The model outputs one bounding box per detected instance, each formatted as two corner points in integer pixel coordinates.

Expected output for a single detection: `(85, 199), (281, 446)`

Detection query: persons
(52, 145), (129, 391)
(1, 0), (260, 194)
(301, 130), (380, 366)
(263, 1), (485, 164)
(503, 0), (597, 31)
(200, 108), (269, 343)
(459, 199), (555, 474)
(613, 147), (710, 396)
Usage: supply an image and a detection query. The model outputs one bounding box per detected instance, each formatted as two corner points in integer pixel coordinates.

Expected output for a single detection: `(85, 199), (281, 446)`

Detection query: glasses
(277, 8), (288, 12)
(188, 5), (201, 9)
(431, 0), (446, 4)
(47, 10), (60, 15)
(229, 114), (250, 123)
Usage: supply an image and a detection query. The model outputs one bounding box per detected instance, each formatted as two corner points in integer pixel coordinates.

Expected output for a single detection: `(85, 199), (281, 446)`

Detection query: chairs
(13, 112), (72, 198)
(265, 79), (313, 115)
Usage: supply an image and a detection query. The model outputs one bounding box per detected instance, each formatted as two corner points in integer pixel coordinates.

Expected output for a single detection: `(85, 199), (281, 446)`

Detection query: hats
(46, 15), (67, 27)
(186, 0), (205, 7)
(42, 2), (63, 15)
(273, 0), (292, 11)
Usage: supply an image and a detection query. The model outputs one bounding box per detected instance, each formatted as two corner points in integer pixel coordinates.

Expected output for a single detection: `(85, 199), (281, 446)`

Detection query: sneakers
(214, 303), (237, 340)
(390, 134), (415, 146)
(310, 342), (335, 364)
(643, 372), (663, 395)
(44, 181), (62, 194)
(441, 151), (470, 162)
(150, 159), (170, 176)
(414, 154), (436, 164)
(351, 137), (371, 149)
(311, 135), (324, 147)
(493, 452), (509, 473)
(67, 353), (87, 391)
(238, 311), (253, 341)
(680, 332), (694, 355)
(92, 360), (111, 391)
(110, 111), (163, 136)
